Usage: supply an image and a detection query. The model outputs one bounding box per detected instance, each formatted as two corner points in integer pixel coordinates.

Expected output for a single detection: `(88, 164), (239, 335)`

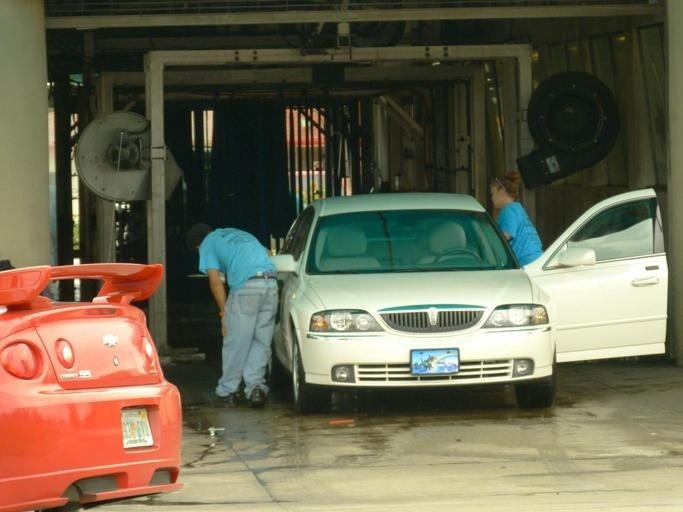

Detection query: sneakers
(195, 390), (238, 409)
(247, 386), (265, 407)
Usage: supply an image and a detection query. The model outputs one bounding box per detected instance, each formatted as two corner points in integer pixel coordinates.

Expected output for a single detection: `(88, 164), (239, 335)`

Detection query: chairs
(319, 222), (381, 271)
(415, 223), (480, 265)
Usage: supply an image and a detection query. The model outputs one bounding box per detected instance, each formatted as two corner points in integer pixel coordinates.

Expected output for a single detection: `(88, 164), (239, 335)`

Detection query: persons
(485, 172), (544, 268)
(180, 218), (279, 408)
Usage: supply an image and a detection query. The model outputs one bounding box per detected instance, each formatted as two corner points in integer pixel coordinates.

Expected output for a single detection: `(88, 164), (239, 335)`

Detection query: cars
(0, 260), (185, 511)
(270, 186), (667, 412)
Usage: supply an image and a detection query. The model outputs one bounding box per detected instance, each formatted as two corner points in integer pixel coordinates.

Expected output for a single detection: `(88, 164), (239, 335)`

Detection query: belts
(247, 273), (278, 282)
